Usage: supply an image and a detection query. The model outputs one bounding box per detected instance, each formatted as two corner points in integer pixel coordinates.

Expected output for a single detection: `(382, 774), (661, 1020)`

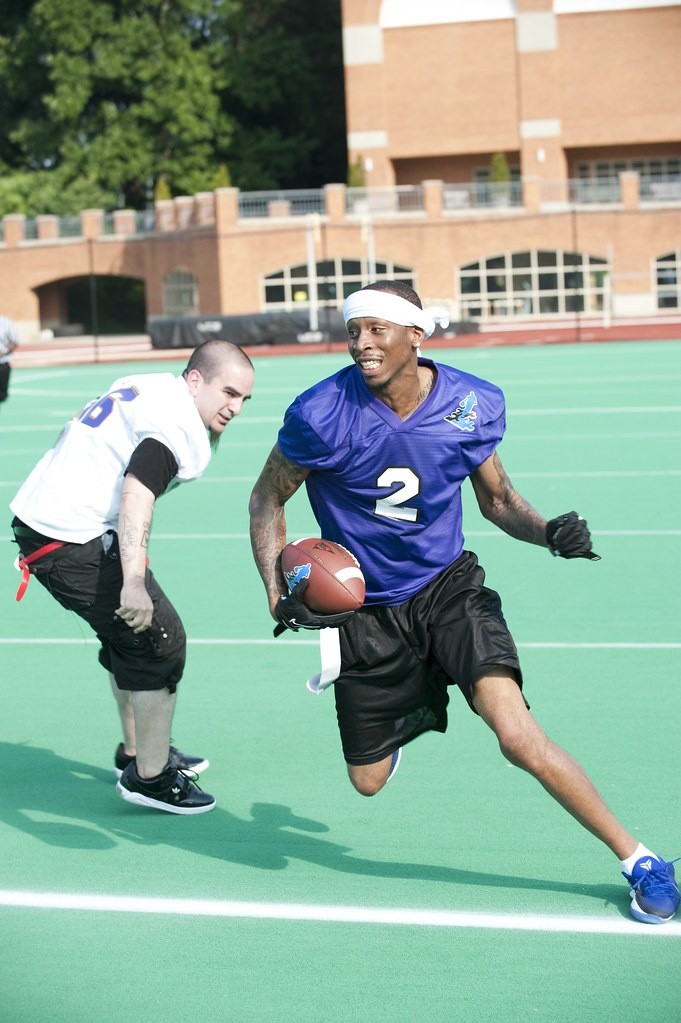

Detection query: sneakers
(620, 854), (680, 924)
(116, 742), (210, 779)
(387, 747), (402, 783)
(115, 760), (215, 813)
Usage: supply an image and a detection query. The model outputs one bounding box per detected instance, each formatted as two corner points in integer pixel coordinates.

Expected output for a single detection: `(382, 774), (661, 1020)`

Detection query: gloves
(273, 578), (355, 637)
(544, 511), (601, 560)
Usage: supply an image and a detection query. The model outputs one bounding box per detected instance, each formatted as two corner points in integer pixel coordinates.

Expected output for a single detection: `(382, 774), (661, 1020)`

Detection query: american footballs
(280, 536), (366, 615)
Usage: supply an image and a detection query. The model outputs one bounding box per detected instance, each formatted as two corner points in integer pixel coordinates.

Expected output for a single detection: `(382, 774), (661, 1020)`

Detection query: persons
(1, 315), (19, 406)
(249, 278), (681, 924)
(9, 340), (257, 814)
